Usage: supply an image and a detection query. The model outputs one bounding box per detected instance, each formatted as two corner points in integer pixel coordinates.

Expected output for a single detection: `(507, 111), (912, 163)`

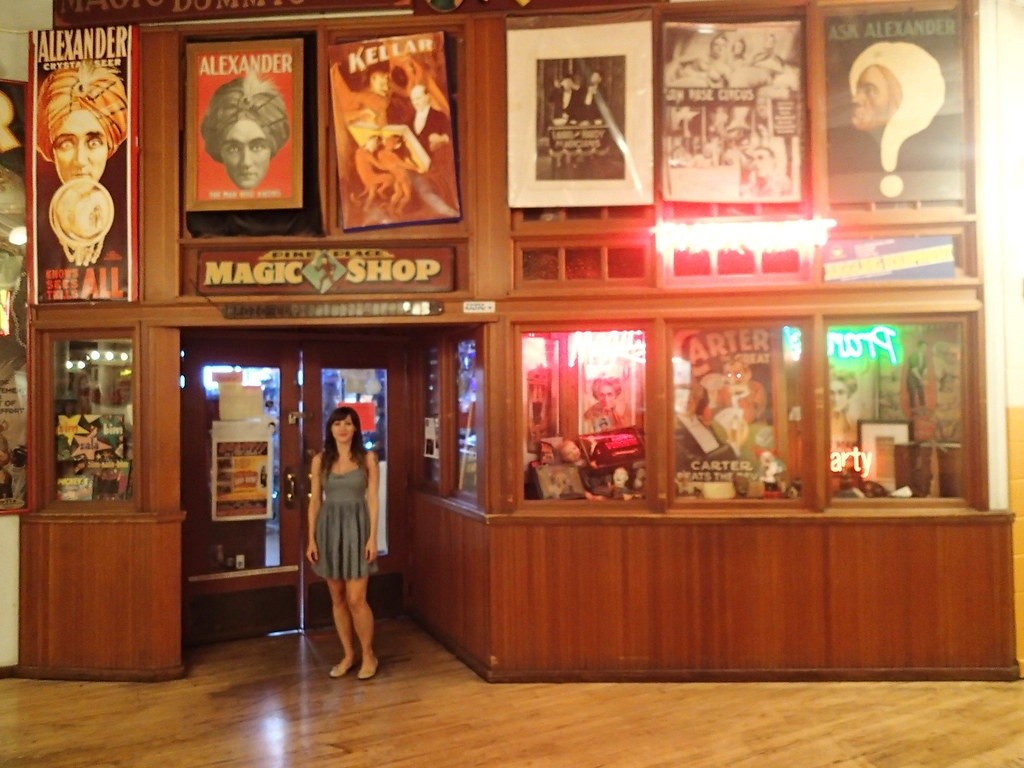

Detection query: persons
(907, 340), (928, 414)
(558, 441), (585, 470)
(548, 73), (604, 126)
(584, 374), (623, 435)
(347, 70), (450, 164)
(306, 407), (380, 678)
(829, 364), (862, 437)
(664, 31), (787, 197)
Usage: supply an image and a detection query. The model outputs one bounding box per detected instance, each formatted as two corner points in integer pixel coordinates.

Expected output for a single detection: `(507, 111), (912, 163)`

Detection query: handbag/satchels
(529, 460), (587, 500)
(577, 425), (647, 475)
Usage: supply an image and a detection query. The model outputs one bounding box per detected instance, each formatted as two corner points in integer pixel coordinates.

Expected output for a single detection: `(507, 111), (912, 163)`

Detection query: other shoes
(328, 661), (359, 678)
(357, 658), (378, 679)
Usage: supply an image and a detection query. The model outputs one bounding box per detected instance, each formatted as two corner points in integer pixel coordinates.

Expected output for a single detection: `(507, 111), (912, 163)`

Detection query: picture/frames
(180, 32), (316, 217)
(654, 6), (815, 220)
